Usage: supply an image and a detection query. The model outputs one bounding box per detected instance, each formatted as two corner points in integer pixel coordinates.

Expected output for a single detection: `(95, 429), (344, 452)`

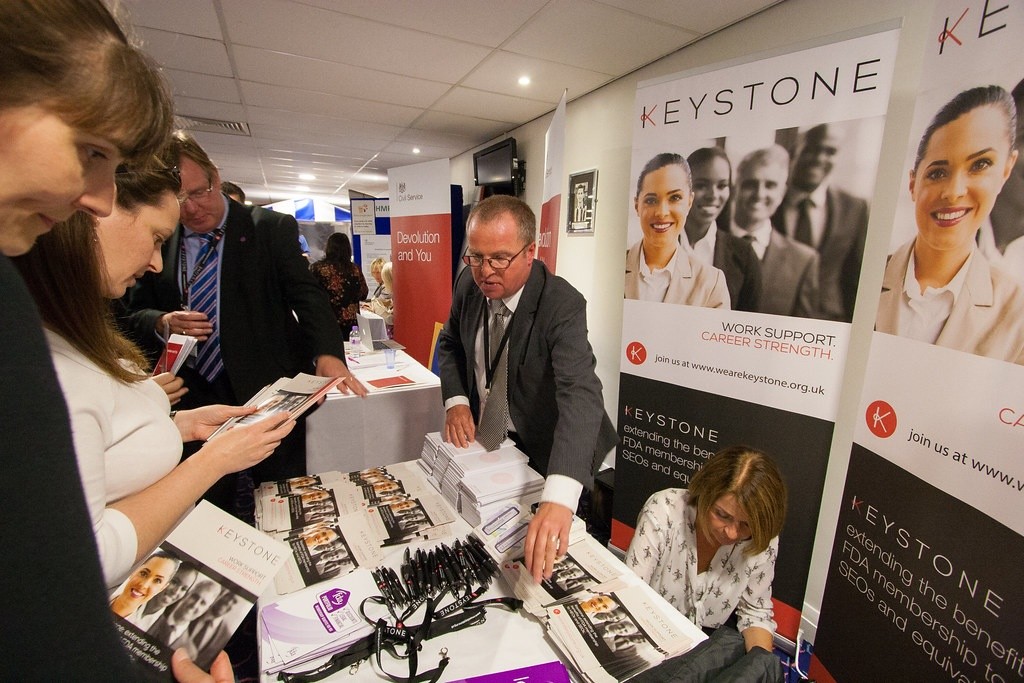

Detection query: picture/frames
(565, 168), (597, 237)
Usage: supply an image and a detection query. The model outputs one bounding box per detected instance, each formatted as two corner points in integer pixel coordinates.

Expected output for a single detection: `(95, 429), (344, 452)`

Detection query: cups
(384, 349), (396, 369)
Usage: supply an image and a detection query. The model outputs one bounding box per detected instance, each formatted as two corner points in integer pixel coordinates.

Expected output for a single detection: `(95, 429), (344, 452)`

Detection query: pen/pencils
(371, 533), (501, 610)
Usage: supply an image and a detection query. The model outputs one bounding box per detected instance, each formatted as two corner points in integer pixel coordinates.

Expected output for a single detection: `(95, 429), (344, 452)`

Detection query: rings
(182, 330), (185, 335)
(549, 537), (557, 541)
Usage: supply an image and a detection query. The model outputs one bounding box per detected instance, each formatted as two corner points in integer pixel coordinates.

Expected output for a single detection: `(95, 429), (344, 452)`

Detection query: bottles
(349, 326), (361, 358)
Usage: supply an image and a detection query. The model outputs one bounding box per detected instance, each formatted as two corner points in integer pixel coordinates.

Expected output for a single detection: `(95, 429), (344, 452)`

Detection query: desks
(305, 343), (445, 475)
(252, 454), (707, 683)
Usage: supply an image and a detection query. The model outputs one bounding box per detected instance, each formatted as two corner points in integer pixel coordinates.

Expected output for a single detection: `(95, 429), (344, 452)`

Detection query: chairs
(427, 321), (443, 377)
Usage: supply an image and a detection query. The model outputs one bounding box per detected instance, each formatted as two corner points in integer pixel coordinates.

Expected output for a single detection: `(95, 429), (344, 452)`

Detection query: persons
(874, 81), (1024, 364)
(115, 131), (367, 526)
(0, 1), (233, 683)
(311, 232), (368, 341)
(254, 394), (305, 414)
(360, 466), (425, 532)
(543, 559), (647, 658)
(299, 235), (313, 259)
(729, 121), (868, 319)
(679, 149), (760, 312)
(290, 467), (350, 582)
(627, 153), (732, 311)
(110, 551), (237, 674)
(221, 182), (245, 203)
(440, 195), (620, 584)
(625, 446), (787, 653)
(361, 256), (392, 324)
(37, 154), (297, 592)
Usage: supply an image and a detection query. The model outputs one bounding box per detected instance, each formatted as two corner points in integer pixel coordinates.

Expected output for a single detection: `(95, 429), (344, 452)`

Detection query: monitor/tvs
(472, 137), (517, 186)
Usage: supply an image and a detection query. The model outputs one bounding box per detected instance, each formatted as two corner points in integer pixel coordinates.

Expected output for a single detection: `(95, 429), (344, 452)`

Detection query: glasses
(116, 163), (182, 186)
(187, 177), (212, 202)
(461, 245), (528, 270)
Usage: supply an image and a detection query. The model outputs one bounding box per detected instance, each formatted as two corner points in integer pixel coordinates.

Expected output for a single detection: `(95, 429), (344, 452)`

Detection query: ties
(605, 629), (609, 634)
(478, 299), (512, 453)
(324, 564), (336, 571)
(566, 581), (578, 588)
(615, 639), (629, 648)
(191, 619), (213, 649)
(311, 515), (322, 519)
(168, 626), (176, 635)
(405, 522), (415, 527)
(186, 229), (225, 384)
(742, 234), (756, 244)
(794, 198), (815, 247)
(556, 575), (560, 580)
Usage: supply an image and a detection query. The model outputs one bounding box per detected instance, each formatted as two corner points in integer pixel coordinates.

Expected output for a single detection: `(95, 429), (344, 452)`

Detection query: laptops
(354, 313), (406, 352)
